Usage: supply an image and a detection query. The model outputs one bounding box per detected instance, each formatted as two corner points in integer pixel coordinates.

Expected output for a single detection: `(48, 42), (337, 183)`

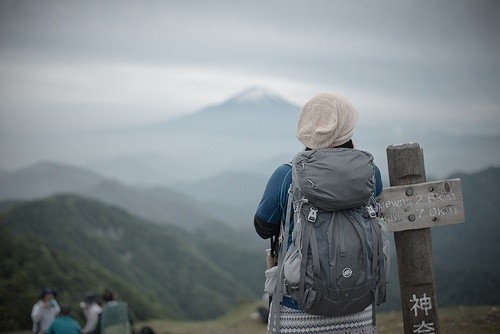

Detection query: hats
(295, 92), (360, 150)
(39, 286), (55, 298)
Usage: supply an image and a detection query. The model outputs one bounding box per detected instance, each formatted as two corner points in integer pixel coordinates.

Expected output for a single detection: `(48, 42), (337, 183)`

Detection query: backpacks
(268, 147), (389, 334)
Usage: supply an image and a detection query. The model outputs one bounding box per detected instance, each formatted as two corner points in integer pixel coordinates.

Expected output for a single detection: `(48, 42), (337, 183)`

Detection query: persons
(253, 92), (384, 334)
(30, 286), (142, 334)
